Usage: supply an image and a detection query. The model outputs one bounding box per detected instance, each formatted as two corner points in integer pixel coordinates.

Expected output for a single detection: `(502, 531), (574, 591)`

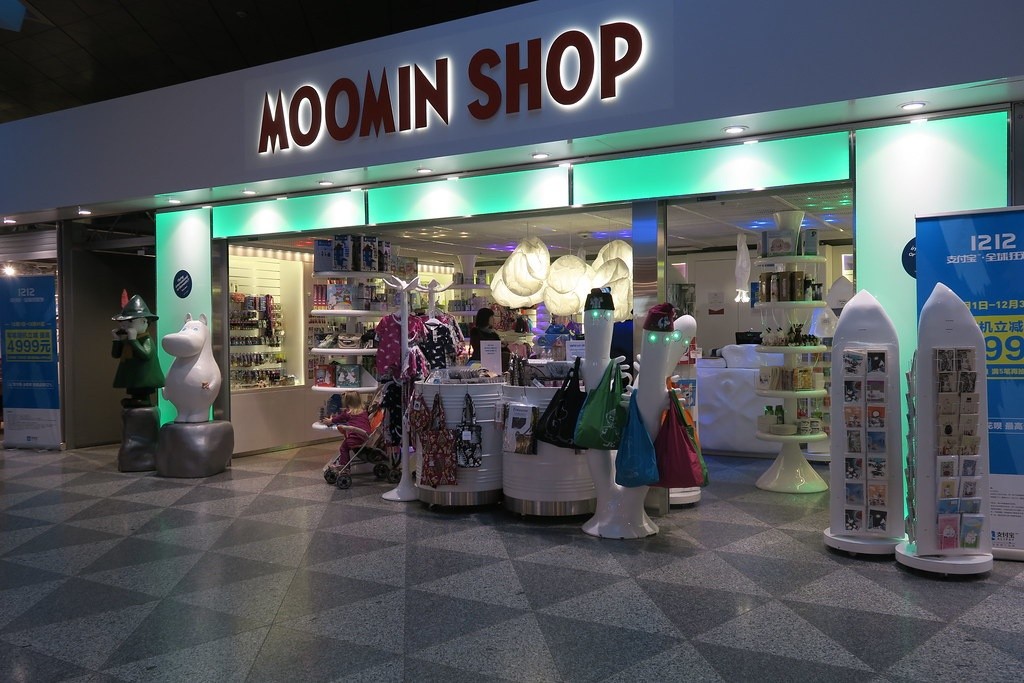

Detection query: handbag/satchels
(573, 359), (627, 450)
(534, 357), (588, 450)
(454, 393), (482, 468)
(615, 389), (659, 488)
(411, 394), (458, 488)
(646, 389), (709, 489)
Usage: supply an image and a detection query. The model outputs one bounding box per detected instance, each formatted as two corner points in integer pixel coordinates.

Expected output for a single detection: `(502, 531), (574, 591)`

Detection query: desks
(414, 380), (506, 514)
(502, 384), (599, 525)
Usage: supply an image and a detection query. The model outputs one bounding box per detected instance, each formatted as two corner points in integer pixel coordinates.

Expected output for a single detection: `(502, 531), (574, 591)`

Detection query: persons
(324, 391), (370, 473)
(111, 295), (165, 408)
(472, 309), (500, 360)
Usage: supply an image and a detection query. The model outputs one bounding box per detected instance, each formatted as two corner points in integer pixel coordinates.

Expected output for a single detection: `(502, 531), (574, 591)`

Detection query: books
(937, 348), (985, 549)
(843, 348), (887, 537)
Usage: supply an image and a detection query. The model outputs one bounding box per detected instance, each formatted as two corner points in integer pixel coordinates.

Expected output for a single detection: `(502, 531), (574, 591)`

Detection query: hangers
(424, 308), (445, 325)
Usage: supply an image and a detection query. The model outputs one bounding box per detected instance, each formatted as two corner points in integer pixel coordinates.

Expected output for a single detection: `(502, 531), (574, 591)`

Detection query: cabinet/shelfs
(310, 272), (391, 431)
(754, 257), (830, 493)
(670, 303), (701, 506)
(230, 291), (304, 391)
(450, 254), (489, 342)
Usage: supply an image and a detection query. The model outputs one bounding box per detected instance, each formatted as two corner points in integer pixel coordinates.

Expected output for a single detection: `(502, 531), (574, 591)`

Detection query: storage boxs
(794, 368), (812, 391)
(756, 229), (823, 257)
(502, 402), (539, 455)
(313, 234), (419, 388)
(448, 270), (489, 312)
(566, 340), (587, 360)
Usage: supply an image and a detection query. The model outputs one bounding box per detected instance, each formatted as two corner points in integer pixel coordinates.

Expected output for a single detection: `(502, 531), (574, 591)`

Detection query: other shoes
(330, 464), (350, 475)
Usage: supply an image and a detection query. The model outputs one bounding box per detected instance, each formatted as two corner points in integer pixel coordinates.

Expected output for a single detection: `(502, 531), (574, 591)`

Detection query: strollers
(311, 381), (402, 489)
